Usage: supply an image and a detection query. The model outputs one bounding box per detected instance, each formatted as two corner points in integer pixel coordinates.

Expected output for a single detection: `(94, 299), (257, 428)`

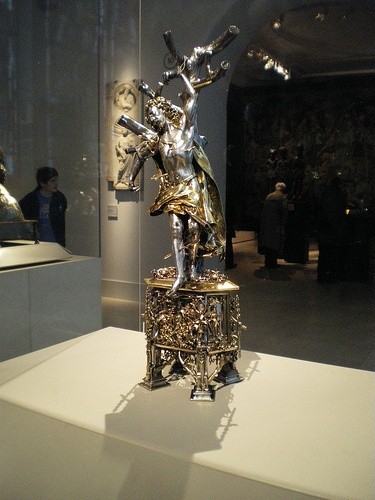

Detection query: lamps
(247, 4), (329, 80)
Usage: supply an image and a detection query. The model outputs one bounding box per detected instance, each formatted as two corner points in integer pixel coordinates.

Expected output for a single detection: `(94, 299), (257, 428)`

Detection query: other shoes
(227, 264), (237, 268)
(268, 264), (280, 268)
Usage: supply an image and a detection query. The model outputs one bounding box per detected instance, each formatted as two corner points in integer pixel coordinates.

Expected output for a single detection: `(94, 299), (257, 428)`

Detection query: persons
(17, 166), (68, 248)
(255, 182), (288, 269)
(127, 64), (227, 298)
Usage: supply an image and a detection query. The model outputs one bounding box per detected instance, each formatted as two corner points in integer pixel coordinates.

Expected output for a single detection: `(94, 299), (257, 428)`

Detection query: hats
(275, 182), (286, 189)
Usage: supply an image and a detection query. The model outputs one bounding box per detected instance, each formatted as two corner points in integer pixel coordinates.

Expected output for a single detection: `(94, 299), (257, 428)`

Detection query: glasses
(47, 179), (58, 182)
